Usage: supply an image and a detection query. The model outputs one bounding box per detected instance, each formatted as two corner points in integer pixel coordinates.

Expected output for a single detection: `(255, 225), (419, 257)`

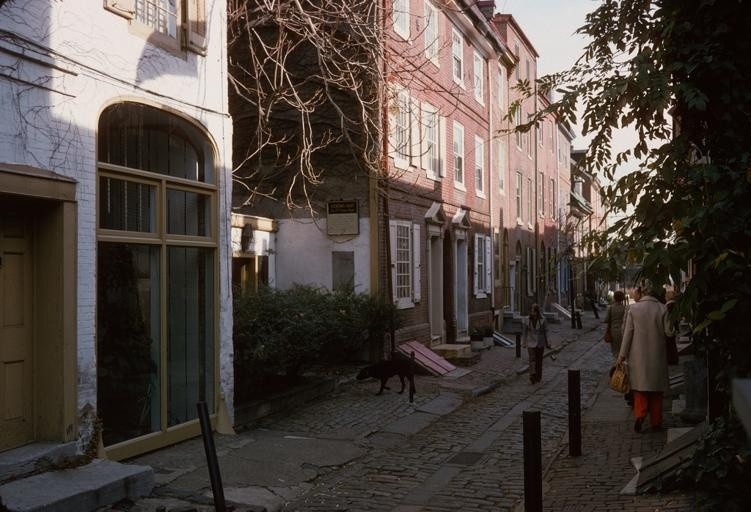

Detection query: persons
(616, 278), (677, 433)
(662, 290), (680, 365)
(605, 290), (627, 356)
(522, 303), (553, 384)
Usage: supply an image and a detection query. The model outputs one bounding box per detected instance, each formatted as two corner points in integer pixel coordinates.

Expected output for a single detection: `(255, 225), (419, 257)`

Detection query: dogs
(355, 359), (417, 396)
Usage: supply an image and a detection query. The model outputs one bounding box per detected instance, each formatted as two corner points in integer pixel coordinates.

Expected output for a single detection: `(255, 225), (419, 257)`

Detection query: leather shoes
(632, 418), (642, 432)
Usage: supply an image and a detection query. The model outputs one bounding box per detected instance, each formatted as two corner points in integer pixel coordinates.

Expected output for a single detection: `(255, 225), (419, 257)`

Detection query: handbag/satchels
(604, 327), (613, 342)
(661, 314), (680, 365)
(610, 360), (630, 393)
